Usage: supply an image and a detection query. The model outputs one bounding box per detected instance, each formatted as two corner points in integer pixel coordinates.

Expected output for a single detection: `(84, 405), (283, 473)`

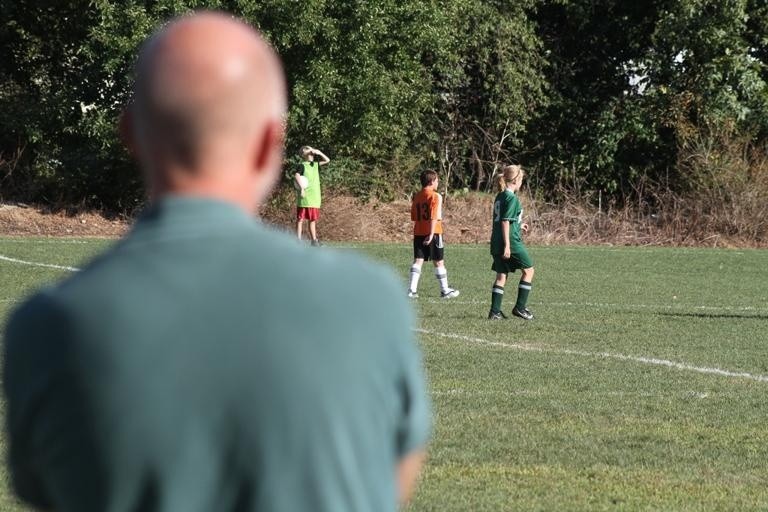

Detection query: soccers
(294, 175), (308, 190)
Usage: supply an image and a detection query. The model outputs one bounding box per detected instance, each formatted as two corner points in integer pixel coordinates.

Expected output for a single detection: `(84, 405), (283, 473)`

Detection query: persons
(0, 12), (434, 512)
(483, 164), (535, 321)
(404, 169), (462, 300)
(292, 146), (331, 247)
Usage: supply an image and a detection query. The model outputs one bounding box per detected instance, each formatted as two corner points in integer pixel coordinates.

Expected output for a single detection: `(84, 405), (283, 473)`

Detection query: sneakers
(407, 289), (419, 299)
(487, 310), (508, 321)
(440, 289), (459, 300)
(512, 305), (535, 320)
(311, 239), (324, 248)
